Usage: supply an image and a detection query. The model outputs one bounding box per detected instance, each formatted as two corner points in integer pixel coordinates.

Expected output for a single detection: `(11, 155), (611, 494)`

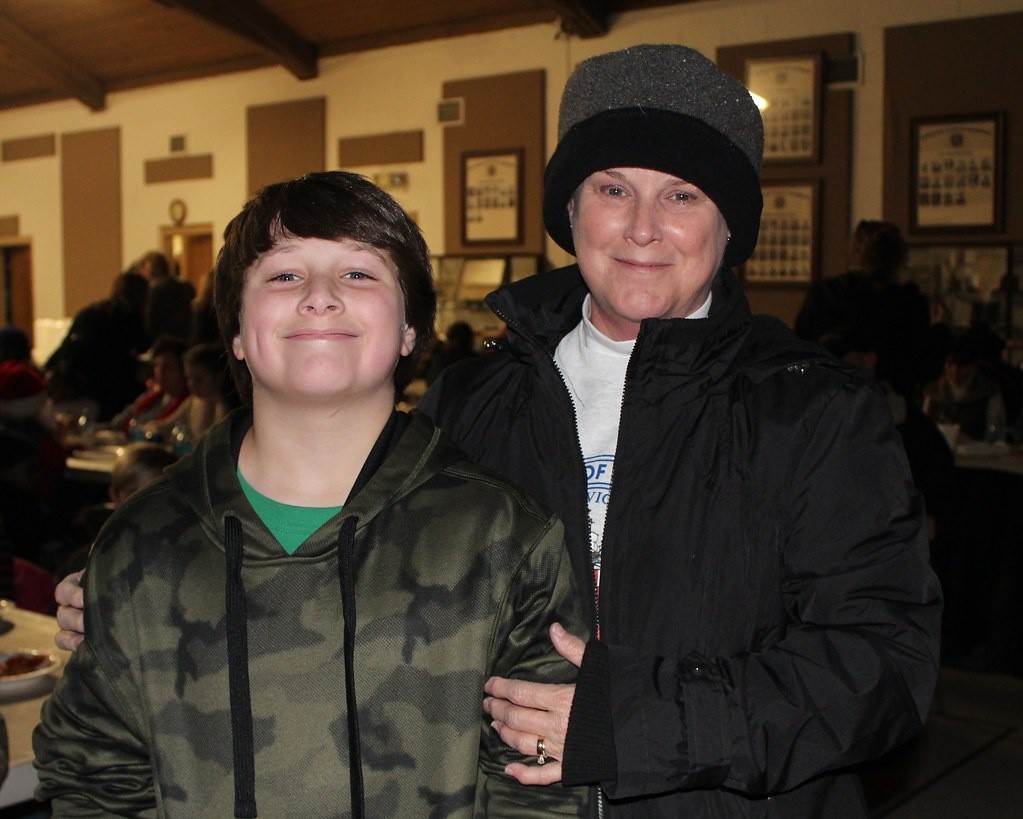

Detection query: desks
(0, 600), (73, 808)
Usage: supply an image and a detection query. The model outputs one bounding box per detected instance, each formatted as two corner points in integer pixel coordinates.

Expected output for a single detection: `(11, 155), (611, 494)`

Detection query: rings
(537, 735), (548, 765)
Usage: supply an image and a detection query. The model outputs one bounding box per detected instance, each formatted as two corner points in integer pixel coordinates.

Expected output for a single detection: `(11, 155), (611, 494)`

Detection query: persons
(427, 320), (483, 387)
(796, 221), (1023, 539)
(30, 169), (593, 818)
(54, 41), (943, 819)
(0, 250), (229, 617)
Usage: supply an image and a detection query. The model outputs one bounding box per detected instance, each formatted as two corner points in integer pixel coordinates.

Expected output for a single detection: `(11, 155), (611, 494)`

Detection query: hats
(541, 44), (763, 268)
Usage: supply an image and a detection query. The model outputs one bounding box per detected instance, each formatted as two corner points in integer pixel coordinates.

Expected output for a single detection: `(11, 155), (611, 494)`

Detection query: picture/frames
(742, 49), (826, 164)
(461, 145), (527, 247)
(737, 175), (826, 290)
(909, 112), (1003, 234)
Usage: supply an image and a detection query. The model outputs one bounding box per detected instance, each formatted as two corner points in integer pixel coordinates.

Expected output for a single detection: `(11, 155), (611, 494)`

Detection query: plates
(0, 648), (64, 692)
(956, 446), (1010, 459)
